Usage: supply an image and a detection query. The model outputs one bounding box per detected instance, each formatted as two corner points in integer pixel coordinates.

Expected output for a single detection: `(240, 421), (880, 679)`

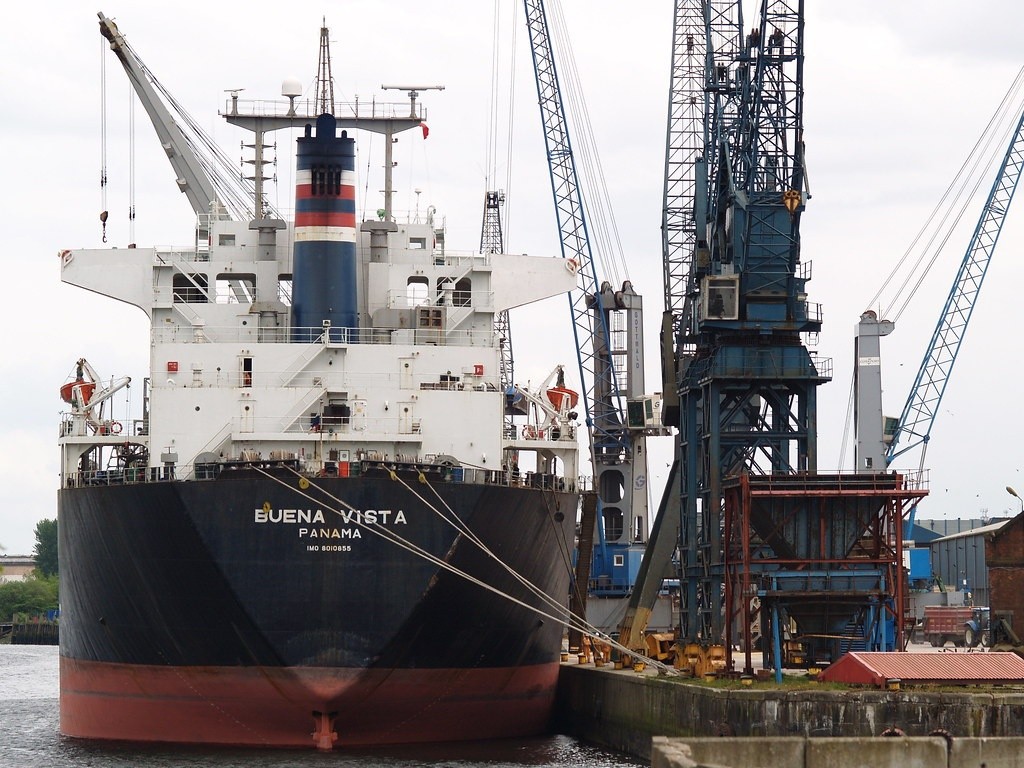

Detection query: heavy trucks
(922, 605), (991, 648)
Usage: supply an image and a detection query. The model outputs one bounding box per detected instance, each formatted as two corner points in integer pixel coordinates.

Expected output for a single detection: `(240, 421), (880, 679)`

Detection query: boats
(54, 8), (583, 755)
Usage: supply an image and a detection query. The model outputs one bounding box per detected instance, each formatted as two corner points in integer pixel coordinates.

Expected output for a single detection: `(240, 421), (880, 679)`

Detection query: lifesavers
(522, 427), (534, 438)
(111, 422), (123, 434)
